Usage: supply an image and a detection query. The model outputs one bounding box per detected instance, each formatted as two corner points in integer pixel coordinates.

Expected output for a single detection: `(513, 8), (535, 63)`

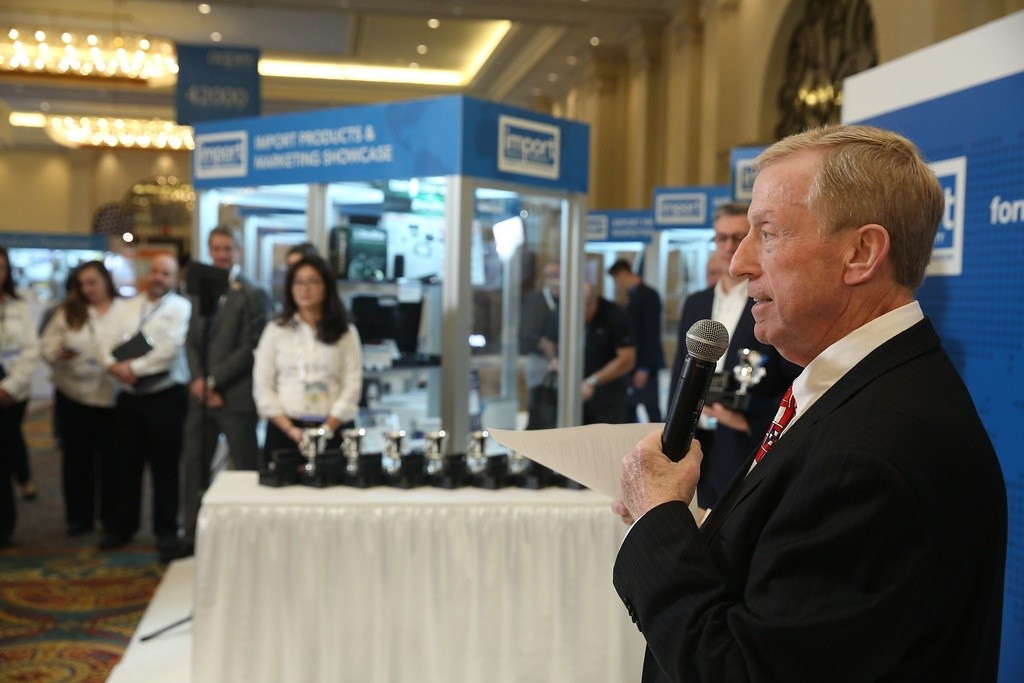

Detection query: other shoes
(155, 527), (176, 549)
(19, 483), (37, 501)
(98, 530), (130, 551)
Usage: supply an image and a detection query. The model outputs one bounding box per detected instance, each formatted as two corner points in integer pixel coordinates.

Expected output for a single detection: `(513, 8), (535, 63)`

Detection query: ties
(746, 386), (798, 473)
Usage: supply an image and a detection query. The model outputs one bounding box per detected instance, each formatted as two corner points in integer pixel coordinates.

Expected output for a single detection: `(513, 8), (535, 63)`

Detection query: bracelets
(283, 423), (295, 435)
(588, 375), (598, 389)
(205, 374), (218, 392)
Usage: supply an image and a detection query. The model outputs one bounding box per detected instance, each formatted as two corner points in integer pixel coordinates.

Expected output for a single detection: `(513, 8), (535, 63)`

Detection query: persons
(614, 123), (1008, 683)
(0, 225), (363, 551)
(524, 200), (805, 512)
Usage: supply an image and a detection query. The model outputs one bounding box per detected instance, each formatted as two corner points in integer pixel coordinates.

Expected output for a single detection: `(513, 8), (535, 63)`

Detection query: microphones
(664, 320), (729, 464)
